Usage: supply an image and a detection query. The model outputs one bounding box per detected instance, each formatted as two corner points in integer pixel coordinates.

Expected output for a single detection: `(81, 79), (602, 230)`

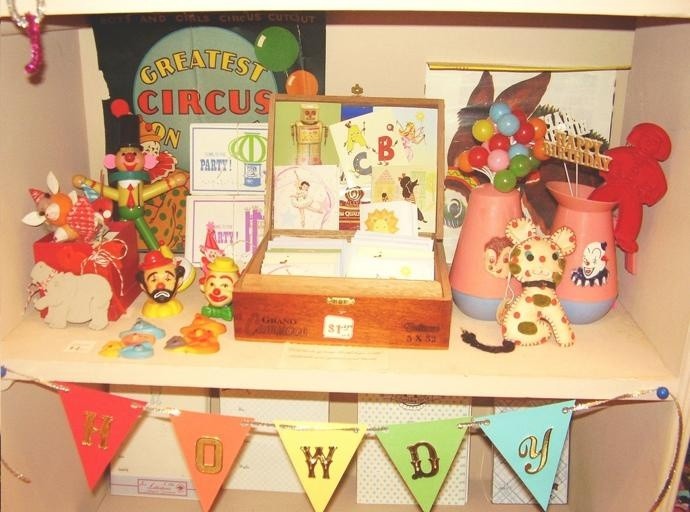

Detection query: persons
(136, 249), (183, 318)
(198, 257), (241, 322)
(287, 169), (326, 229)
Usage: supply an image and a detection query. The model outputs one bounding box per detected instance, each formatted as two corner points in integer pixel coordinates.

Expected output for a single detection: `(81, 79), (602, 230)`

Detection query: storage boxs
(232, 90), (455, 351)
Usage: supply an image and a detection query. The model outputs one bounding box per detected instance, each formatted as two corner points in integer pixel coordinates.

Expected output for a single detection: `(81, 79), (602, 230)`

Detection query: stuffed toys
(459, 213), (578, 355)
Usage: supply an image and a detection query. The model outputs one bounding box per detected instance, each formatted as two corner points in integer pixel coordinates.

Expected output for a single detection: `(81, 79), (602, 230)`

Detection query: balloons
(253, 23), (302, 74)
(284, 68), (321, 97)
